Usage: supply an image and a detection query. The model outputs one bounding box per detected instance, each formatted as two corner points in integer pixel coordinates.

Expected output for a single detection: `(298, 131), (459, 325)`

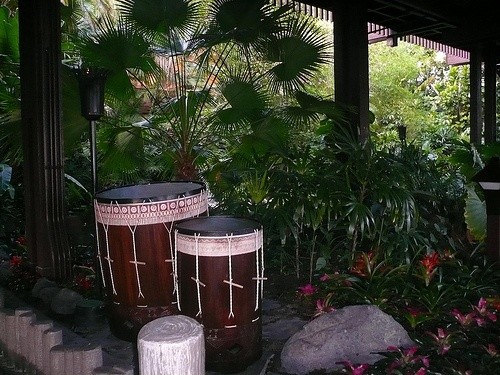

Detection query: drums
(167, 214), (264, 368)
(93, 181), (209, 342)
